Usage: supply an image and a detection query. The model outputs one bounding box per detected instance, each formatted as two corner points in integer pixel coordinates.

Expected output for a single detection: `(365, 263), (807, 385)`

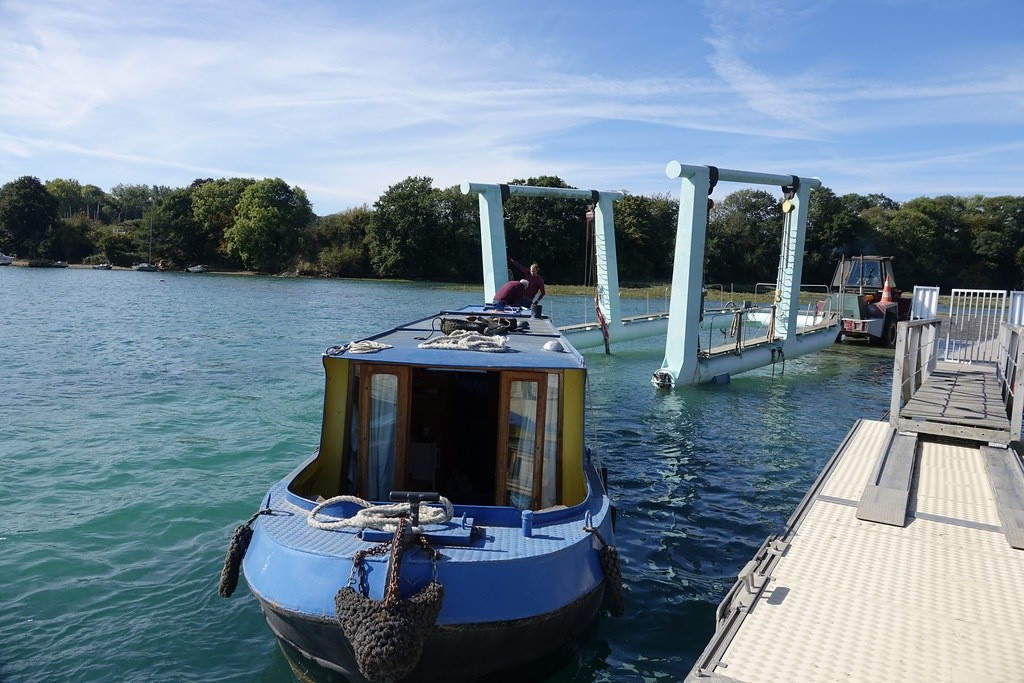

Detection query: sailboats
(130, 217), (157, 272)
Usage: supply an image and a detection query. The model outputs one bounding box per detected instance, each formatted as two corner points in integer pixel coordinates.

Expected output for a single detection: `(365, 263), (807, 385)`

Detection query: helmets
(516, 320), (531, 331)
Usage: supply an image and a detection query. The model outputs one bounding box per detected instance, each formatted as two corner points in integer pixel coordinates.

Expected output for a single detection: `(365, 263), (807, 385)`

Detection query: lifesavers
(440, 318), (488, 334)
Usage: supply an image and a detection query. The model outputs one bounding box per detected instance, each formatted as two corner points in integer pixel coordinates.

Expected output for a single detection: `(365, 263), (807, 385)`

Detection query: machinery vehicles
(816, 255), (912, 348)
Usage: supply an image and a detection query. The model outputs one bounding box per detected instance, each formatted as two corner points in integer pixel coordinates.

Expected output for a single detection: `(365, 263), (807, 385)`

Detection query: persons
(508, 256), (545, 308)
(493, 279), (529, 305)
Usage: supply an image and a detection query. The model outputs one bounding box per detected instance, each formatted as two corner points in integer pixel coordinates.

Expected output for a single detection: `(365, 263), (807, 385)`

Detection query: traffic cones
(879, 275), (893, 304)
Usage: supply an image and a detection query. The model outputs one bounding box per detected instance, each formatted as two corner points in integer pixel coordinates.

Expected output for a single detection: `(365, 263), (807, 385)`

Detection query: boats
(91, 264), (112, 270)
(185, 265), (215, 273)
(0, 253), (14, 265)
(241, 265), (616, 683)
(52, 260), (69, 268)
(155, 259), (174, 270)
(747, 307), (822, 328)
